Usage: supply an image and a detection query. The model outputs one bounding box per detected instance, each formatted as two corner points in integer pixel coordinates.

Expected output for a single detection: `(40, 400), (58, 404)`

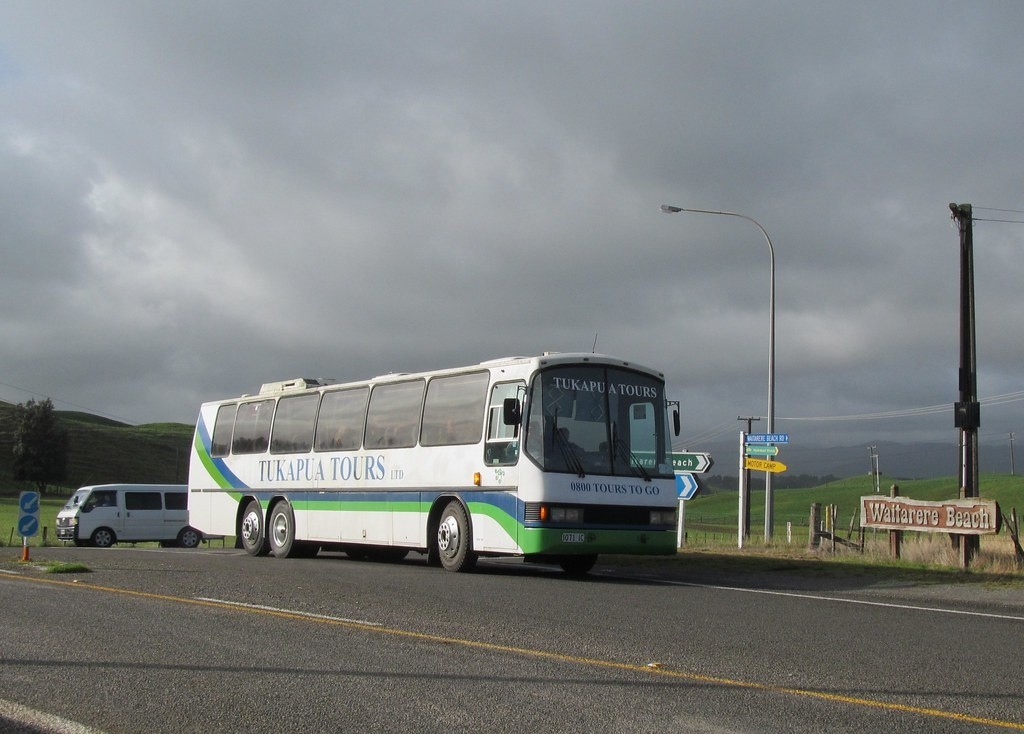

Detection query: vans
(55, 484), (202, 548)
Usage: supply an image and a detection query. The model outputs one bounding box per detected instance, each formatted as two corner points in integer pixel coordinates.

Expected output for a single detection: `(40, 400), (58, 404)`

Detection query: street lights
(662, 206), (775, 544)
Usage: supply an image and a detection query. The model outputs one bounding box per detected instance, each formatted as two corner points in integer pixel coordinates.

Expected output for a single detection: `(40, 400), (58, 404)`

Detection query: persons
(556, 427), (584, 455)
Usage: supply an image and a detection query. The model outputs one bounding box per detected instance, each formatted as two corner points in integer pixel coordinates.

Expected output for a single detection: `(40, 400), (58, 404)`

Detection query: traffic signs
(744, 434), (788, 473)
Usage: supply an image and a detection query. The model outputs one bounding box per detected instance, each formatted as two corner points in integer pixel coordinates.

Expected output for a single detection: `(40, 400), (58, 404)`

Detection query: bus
(187, 335), (680, 574)
